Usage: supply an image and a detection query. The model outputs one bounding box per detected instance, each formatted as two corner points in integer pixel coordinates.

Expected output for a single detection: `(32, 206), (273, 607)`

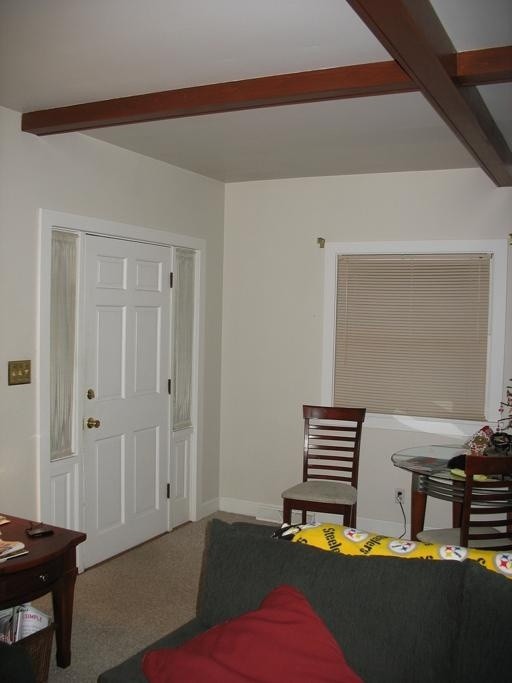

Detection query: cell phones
(28, 527), (53, 539)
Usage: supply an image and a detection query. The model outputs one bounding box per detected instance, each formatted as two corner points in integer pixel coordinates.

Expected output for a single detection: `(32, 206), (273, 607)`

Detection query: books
(0, 539), (29, 563)
(0, 601), (52, 644)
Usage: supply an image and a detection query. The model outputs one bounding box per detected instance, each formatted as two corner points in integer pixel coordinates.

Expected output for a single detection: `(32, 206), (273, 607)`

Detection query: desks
(0, 511), (87, 669)
(390, 441), (511, 551)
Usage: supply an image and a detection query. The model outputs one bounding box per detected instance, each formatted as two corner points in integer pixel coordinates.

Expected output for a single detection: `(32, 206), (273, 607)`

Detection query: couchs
(96, 516), (511, 682)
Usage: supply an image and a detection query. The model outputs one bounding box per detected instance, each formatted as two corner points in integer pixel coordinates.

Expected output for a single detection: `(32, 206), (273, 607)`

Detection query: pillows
(139, 583), (366, 683)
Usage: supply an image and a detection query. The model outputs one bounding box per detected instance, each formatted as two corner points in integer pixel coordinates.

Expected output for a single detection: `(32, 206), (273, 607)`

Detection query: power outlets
(394, 488), (404, 503)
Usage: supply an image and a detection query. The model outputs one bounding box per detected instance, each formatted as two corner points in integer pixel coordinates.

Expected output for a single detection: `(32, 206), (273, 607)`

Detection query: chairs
(280, 403), (366, 528)
(415, 454), (512, 553)
